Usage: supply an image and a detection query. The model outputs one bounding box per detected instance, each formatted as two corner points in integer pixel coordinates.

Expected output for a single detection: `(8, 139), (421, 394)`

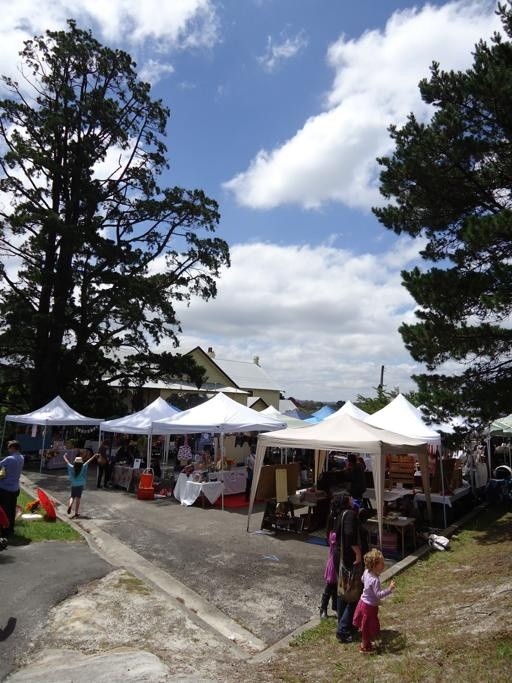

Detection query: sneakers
(336, 623), (359, 642)
(319, 607), (338, 619)
(358, 640), (378, 654)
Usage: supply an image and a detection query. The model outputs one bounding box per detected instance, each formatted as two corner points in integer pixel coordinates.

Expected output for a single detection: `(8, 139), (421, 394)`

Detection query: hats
(73, 456), (85, 464)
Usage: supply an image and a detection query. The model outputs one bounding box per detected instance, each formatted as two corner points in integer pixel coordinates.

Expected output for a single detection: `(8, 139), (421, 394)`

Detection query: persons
(0, 440), (24, 537)
(62, 453), (99, 517)
(202, 446), (214, 465)
(154, 464), (194, 494)
(97, 435), (162, 489)
(320, 454), (396, 652)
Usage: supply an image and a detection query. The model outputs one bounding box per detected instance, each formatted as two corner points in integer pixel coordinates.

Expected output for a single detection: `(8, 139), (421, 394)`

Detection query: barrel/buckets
(141, 468), (154, 488)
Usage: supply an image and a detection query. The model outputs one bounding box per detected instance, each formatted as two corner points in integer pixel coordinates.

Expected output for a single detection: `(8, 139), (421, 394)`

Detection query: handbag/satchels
(335, 561), (364, 604)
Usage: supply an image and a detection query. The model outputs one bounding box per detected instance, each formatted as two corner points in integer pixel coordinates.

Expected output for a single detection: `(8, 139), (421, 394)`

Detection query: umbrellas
(30, 488), (56, 521)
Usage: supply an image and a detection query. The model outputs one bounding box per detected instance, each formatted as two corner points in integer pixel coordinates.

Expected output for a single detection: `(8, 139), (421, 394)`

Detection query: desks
(366, 513), (417, 559)
(365, 479), (471, 529)
(38, 448), (79, 470)
(173, 466), (249, 510)
(109, 463), (140, 492)
